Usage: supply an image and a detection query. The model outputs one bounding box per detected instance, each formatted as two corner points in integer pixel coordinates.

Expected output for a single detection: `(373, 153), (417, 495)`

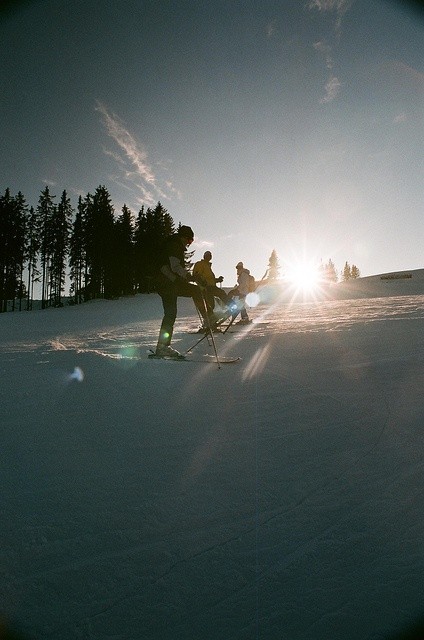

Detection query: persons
(219, 262), (256, 323)
(193, 251), (239, 331)
(137, 226), (224, 358)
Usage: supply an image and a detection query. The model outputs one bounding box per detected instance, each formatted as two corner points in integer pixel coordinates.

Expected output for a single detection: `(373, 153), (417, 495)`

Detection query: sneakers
(156, 343), (180, 356)
(203, 311), (223, 327)
(239, 316), (249, 324)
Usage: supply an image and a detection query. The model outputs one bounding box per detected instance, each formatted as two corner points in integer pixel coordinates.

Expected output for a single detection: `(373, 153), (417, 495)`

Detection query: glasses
(178, 236), (193, 244)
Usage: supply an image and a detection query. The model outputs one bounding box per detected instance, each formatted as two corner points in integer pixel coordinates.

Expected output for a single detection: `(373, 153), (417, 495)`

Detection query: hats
(177, 226), (194, 243)
(204, 251), (211, 261)
(236, 262), (243, 270)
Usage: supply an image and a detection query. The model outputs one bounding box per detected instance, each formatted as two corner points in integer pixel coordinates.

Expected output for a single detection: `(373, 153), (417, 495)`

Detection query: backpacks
(240, 271), (255, 292)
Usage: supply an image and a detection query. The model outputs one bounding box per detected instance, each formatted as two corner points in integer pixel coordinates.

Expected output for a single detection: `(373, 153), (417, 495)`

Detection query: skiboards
(214, 294), (270, 326)
(188, 268), (268, 334)
(148, 307), (241, 363)
